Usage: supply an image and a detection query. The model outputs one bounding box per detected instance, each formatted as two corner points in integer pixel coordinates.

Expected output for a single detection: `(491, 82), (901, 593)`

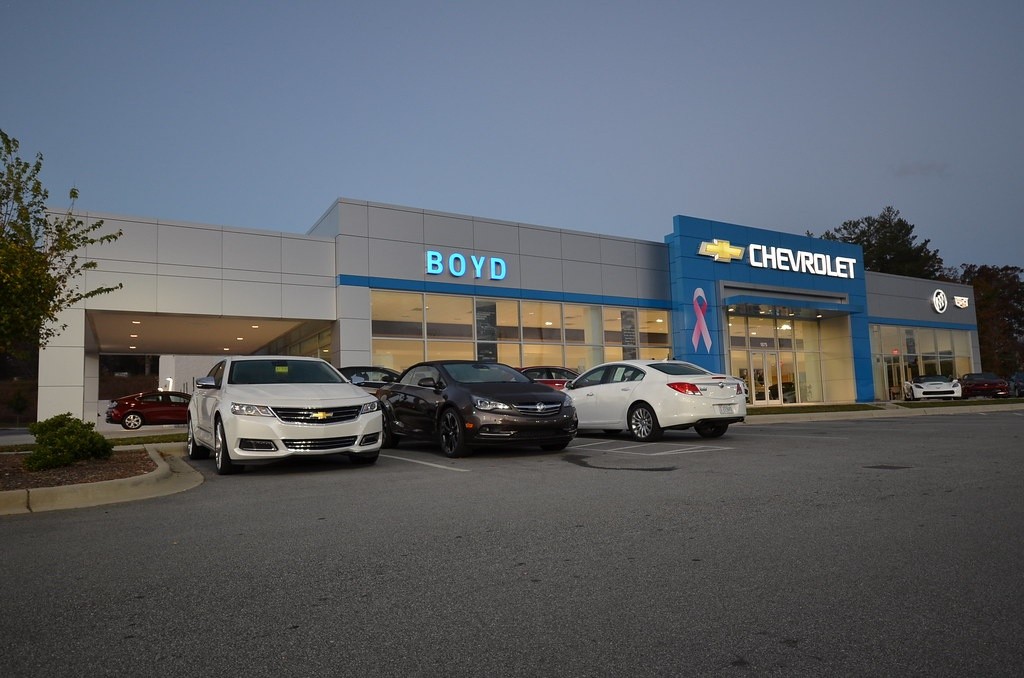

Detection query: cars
(768, 382), (795, 399)
(1008, 372), (1024, 397)
(377, 359), (579, 459)
(105, 390), (192, 430)
(186, 355), (383, 476)
(558, 360), (747, 443)
(337, 366), (403, 396)
(503, 366), (590, 390)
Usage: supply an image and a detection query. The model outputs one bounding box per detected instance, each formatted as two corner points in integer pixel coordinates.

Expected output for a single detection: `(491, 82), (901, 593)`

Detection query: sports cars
(903, 374), (963, 400)
(958, 372), (1009, 399)
